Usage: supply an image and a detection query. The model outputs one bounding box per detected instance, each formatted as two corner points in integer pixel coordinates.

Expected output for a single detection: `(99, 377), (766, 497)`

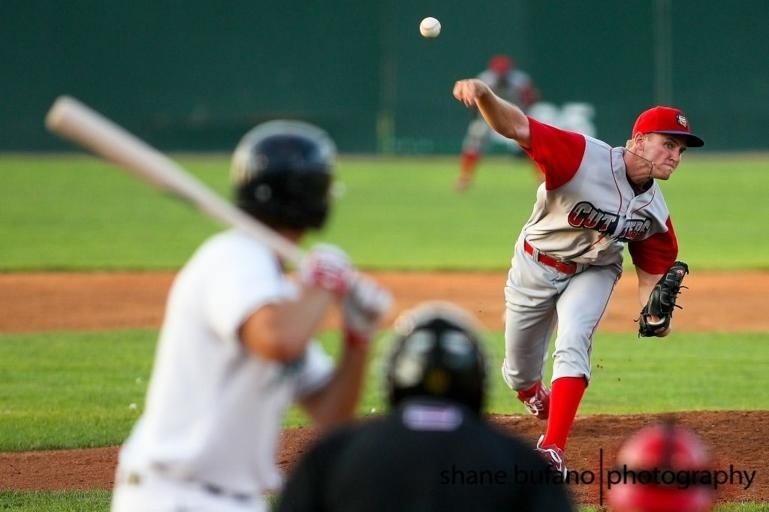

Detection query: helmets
(226, 115), (341, 232)
(383, 297), (490, 423)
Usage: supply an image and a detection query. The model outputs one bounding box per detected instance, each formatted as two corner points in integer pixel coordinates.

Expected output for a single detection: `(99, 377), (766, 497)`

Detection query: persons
(453, 78), (703, 483)
(268, 301), (571, 511)
(455, 60), (535, 192)
(110, 117), (389, 511)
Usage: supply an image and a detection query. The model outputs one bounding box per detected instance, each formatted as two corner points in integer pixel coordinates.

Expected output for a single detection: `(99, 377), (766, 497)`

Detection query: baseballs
(420, 17), (441, 38)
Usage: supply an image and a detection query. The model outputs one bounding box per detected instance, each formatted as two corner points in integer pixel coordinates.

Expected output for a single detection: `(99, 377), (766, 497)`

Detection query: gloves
(291, 240), (353, 298)
(335, 266), (395, 346)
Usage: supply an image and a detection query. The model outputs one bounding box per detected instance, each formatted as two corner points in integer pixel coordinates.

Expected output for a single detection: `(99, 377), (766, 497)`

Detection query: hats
(630, 105), (706, 148)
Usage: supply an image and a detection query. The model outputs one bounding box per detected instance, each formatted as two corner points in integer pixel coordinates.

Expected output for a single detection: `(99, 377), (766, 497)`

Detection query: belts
(524, 240), (577, 276)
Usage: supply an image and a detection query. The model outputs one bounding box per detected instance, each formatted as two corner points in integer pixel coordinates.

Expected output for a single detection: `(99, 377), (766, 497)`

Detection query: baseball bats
(45, 94), (393, 320)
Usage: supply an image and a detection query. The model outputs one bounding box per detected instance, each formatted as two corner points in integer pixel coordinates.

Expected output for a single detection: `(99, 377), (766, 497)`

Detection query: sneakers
(515, 382), (551, 420)
(534, 432), (568, 480)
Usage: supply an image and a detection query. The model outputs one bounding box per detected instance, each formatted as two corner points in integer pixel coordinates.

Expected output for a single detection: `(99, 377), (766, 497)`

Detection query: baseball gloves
(633, 262), (688, 337)
